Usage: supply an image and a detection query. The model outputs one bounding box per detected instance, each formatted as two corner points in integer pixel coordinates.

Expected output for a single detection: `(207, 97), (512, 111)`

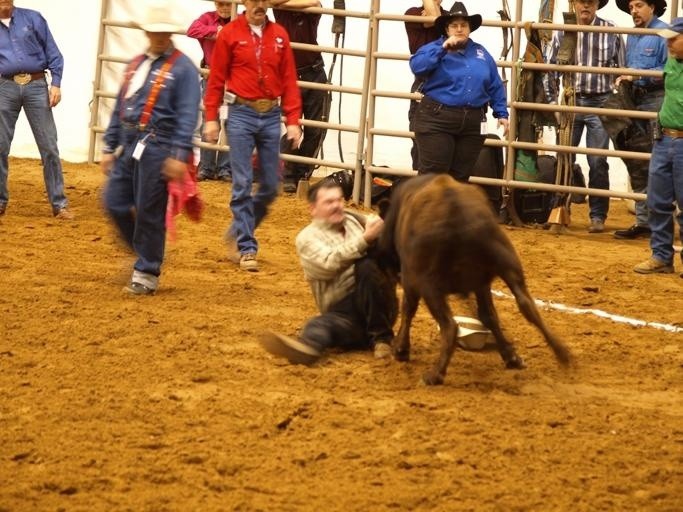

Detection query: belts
(662, 128), (683, 137)
(8, 73), (43, 84)
(576, 93), (601, 99)
(236, 97), (278, 113)
(636, 85), (663, 96)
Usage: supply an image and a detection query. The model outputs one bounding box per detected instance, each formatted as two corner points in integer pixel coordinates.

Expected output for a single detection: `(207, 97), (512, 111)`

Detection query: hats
(656, 17), (683, 39)
(140, 11), (186, 34)
(598, 0), (667, 18)
(436, 2), (481, 34)
(438, 317), (492, 351)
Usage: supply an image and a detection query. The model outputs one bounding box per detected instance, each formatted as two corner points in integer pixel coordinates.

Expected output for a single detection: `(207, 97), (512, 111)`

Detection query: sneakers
(588, 220), (603, 232)
(240, 255), (256, 270)
(283, 176), (296, 192)
(54, 209), (71, 220)
(121, 282), (154, 293)
(634, 258), (675, 275)
(373, 342), (391, 366)
(614, 225), (651, 239)
(261, 333), (319, 365)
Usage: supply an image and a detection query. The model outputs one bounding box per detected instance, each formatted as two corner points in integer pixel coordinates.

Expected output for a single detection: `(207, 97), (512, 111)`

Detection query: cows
(373, 170), (572, 388)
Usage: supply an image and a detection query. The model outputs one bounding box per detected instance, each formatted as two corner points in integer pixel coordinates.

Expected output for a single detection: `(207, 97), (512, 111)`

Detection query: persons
(202, 0), (304, 272)
(633, 15), (683, 278)
(614, 1), (670, 239)
(100, 6), (201, 296)
(186, 0), (244, 182)
(404, 1), (450, 172)
(266, 0), (331, 192)
(542, 0), (627, 232)
(0, 0), (69, 217)
(411, 2), (510, 186)
(257, 181), (398, 366)
